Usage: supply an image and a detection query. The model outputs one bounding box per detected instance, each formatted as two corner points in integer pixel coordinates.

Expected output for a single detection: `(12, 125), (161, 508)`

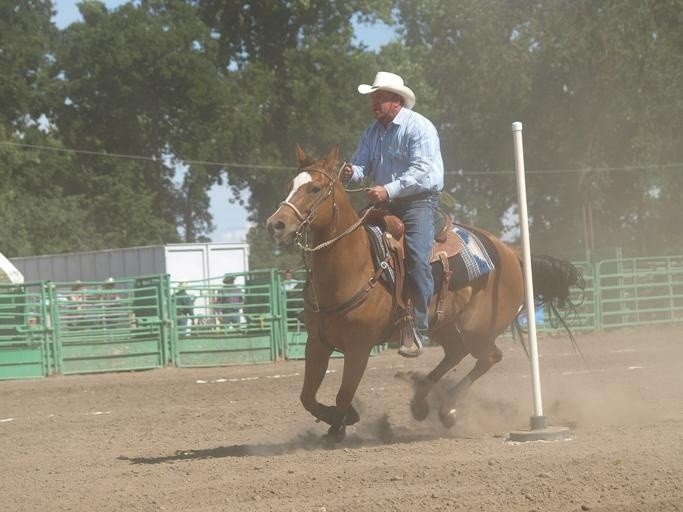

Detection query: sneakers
(399, 336), (419, 356)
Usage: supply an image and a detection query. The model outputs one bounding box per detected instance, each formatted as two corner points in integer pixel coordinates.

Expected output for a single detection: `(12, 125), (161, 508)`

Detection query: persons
(37, 277), (121, 329)
(281, 271), (298, 296)
(171, 283), (194, 338)
(339, 71), (445, 358)
(215, 273), (244, 334)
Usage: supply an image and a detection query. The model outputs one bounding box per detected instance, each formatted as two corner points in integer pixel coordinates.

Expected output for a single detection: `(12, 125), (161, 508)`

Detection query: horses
(266, 142), (588, 449)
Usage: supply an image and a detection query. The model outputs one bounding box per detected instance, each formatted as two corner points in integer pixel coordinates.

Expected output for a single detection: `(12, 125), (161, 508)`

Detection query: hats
(357, 71), (416, 109)
